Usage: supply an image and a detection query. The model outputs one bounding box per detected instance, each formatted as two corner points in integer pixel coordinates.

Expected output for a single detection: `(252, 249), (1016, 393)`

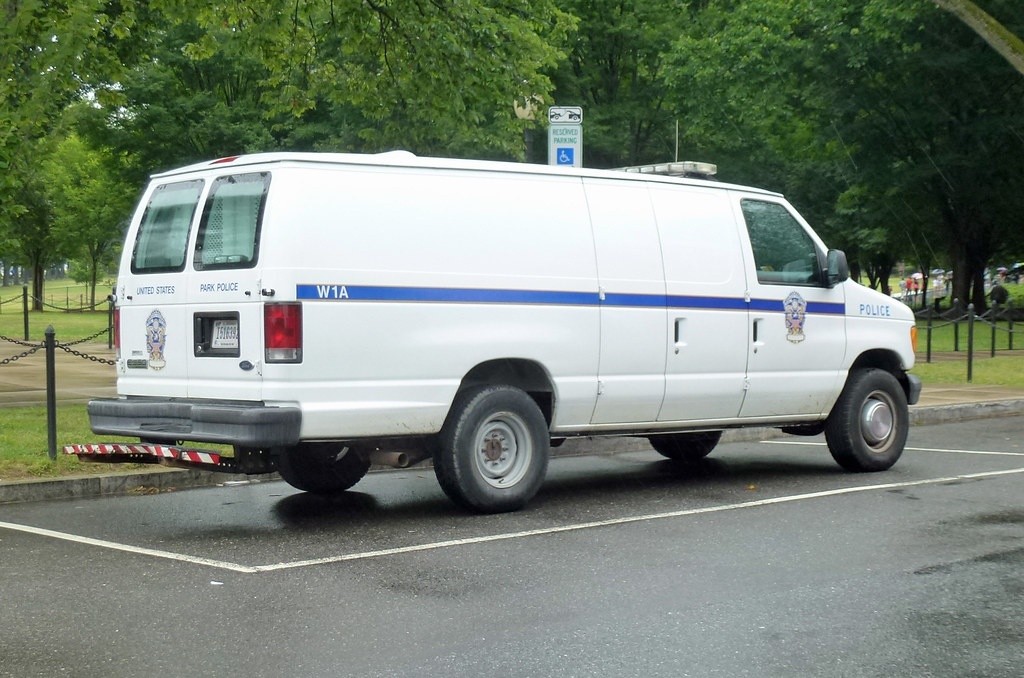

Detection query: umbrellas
(947, 271), (953, 274)
(932, 269), (944, 274)
(911, 273), (928, 279)
(1013, 262), (1024, 268)
(996, 267), (1007, 270)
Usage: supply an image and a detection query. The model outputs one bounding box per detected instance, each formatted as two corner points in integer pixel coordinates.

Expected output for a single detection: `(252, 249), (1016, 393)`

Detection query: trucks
(62, 149), (922, 512)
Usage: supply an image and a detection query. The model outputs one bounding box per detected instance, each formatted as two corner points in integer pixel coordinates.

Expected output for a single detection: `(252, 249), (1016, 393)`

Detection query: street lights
(515, 80), (546, 164)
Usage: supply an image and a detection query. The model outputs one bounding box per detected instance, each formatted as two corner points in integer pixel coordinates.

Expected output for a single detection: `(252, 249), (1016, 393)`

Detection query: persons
(899, 273), (951, 314)
(999, 267), (1024, 283)
